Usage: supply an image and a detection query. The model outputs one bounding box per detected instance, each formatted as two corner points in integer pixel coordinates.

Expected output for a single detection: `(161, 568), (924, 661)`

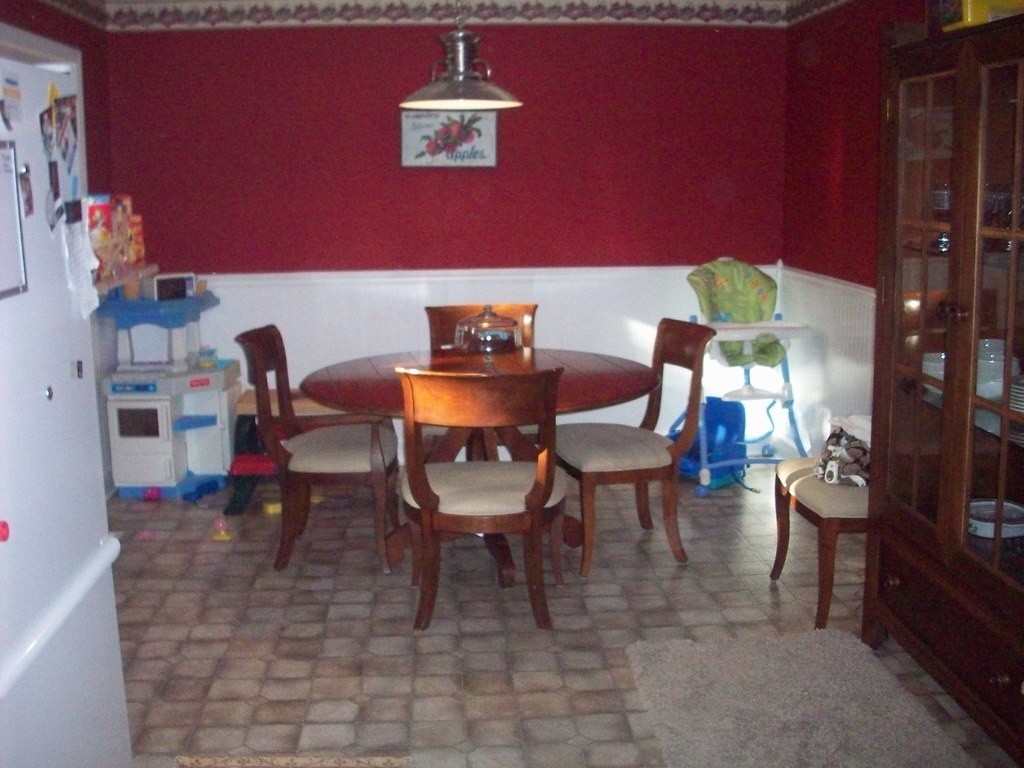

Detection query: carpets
(623, 627), (984, 768)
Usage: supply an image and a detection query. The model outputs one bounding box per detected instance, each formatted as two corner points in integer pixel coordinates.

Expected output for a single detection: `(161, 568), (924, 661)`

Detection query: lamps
(397, 0), (525, 111)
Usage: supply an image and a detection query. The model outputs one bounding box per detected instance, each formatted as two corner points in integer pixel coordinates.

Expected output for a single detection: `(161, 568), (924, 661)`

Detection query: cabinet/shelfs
(860, 12), (1024, 768)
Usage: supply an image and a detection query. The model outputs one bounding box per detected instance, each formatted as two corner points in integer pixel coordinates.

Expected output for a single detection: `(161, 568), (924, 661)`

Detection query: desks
(299, 344), (663, 590)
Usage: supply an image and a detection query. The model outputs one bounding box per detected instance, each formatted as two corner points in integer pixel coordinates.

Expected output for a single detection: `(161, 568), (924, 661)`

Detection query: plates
(1007, 376), (1024, 446)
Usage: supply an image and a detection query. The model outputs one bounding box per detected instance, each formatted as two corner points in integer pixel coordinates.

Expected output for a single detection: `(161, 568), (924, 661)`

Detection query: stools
(767, 455), (868, 629)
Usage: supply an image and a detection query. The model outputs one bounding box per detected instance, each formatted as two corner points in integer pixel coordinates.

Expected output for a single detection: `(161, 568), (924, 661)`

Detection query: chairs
(420, 302), (540, 468)
(234, 324), (402, 577)
(537, 317), (716, 579)
(394, 364), (568, 633)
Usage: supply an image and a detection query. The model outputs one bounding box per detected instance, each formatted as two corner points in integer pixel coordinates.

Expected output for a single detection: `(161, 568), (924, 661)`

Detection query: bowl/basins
(922, 338), (1019, 399)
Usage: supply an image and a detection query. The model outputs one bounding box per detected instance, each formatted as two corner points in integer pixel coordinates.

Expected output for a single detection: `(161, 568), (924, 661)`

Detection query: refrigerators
(0, 22), (132, 768)
